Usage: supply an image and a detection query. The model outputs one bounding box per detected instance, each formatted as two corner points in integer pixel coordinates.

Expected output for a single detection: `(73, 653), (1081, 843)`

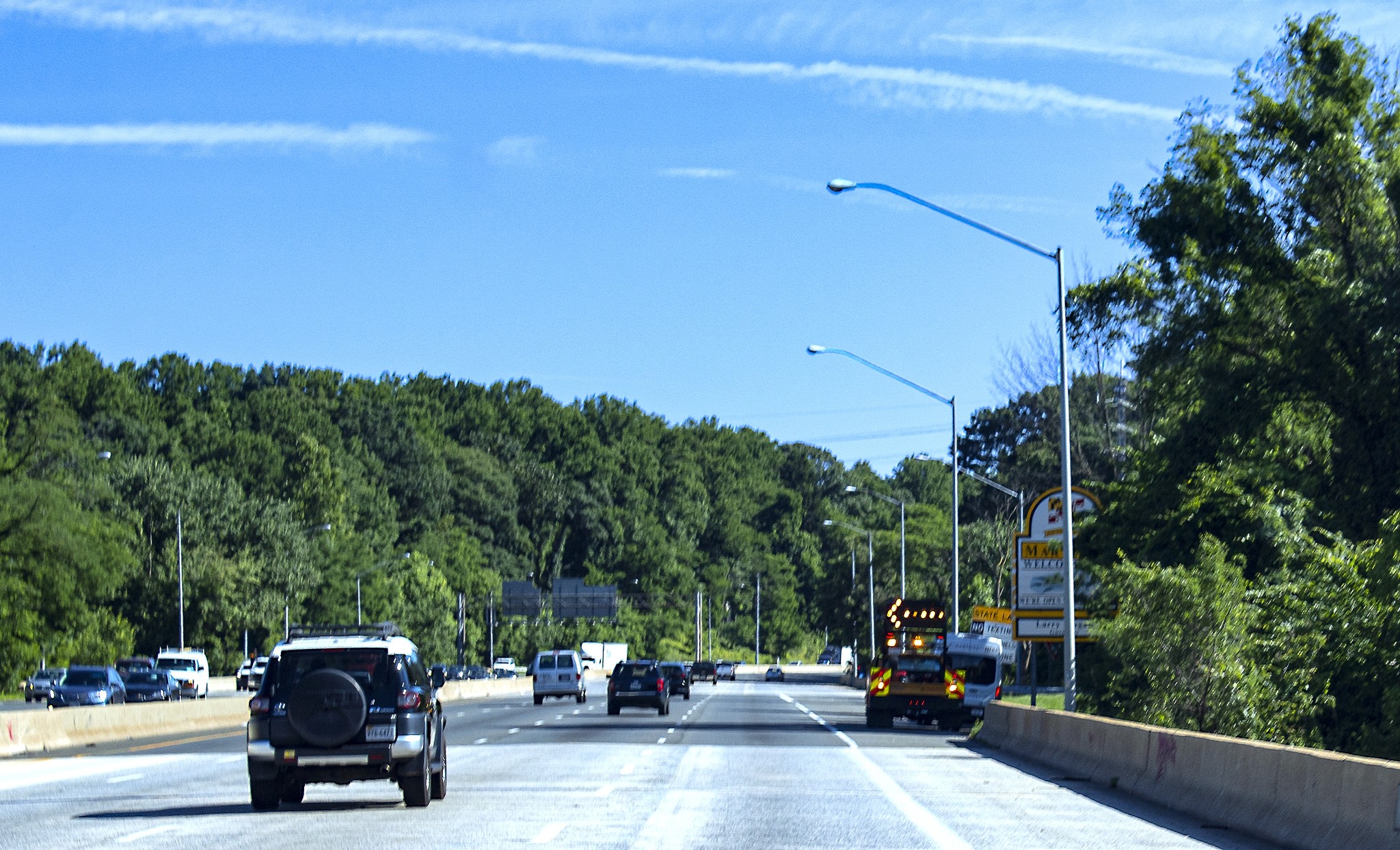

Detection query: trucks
(580, 642), (628, 677)
(817, 646), (841, 665)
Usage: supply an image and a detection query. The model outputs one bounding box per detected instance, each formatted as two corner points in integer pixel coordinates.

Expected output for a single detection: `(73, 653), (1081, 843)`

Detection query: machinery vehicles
(863, 598), (966, 733)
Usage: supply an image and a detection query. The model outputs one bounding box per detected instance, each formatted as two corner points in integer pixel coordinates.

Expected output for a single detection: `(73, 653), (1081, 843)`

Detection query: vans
(153, 646), (209, 701)
(927, 633), (1004, 721)
(528, 650), (589, 706)
(493, 657), (518, 679)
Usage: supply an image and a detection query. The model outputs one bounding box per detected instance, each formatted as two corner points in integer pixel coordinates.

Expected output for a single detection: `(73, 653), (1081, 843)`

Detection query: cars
(660, 658), (746, 701)
(764, 668), (785, 682)
(429, 663), (492, 681)
(234, 654), (269, 692)
(23, 657), (182, 709)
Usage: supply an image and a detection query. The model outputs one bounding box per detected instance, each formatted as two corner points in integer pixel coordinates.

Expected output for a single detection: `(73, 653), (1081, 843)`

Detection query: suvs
(245, 622), (448, 811)
(605, 660), (674, 717)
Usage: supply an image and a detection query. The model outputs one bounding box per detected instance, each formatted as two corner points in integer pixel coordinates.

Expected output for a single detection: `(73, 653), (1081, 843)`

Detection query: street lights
(708, 583), (745, 661)
(357, 551), (411, 626)
(806, 344), (958, 633)
(846, 486), (907, 656)
(284, 524), (332, 639)
(914, 454), (1024, 685)
(827, 177), (1077, 713)
(41, 450), (112, 670)
(822, 519), (875, 662)
